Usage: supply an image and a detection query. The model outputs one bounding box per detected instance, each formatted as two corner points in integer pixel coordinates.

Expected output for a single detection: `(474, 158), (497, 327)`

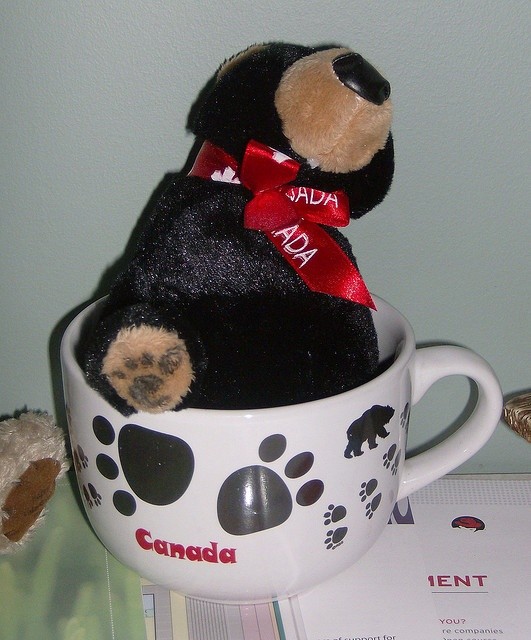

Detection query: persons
(83, 41), (393, 417)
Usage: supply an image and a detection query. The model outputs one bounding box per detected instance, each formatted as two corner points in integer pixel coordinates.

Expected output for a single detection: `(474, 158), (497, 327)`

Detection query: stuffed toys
(1, 409), (72, 556)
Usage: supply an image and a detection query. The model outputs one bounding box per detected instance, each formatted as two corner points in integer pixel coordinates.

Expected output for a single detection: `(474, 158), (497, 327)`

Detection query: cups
(60, 292), (503, 607)
